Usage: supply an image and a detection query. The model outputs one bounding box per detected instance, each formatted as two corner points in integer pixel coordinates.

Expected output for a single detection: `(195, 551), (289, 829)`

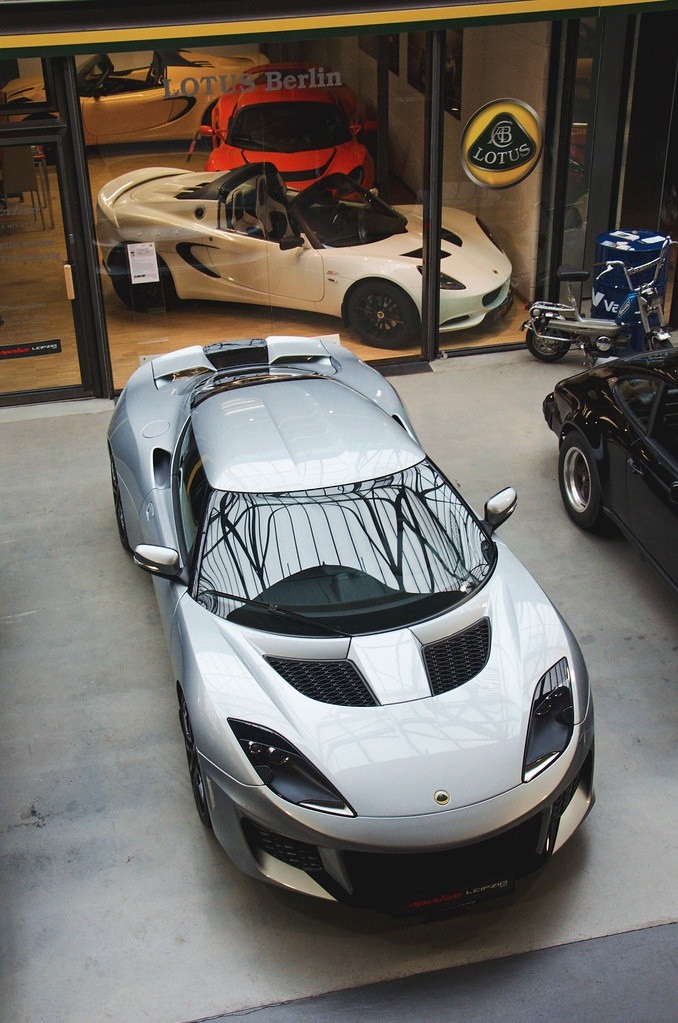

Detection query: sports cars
(107, 331), (594, 930)
(1, 47), (271, 164)
(95, 166), (515, 350)
(198, 62), (380, 209)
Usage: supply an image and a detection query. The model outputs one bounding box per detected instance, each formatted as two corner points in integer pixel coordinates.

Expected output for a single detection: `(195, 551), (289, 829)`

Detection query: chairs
(187, 457), (210, 522)
(145, 52), (165, 89)
(232, 189), (266, 239)
(256, 173), (296, 241)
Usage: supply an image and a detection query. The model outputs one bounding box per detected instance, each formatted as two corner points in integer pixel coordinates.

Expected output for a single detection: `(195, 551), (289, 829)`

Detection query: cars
(538, 346), (677, 603)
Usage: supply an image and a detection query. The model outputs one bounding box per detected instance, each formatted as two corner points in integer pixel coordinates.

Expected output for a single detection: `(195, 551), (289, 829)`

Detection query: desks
(32, 145), (55, 231)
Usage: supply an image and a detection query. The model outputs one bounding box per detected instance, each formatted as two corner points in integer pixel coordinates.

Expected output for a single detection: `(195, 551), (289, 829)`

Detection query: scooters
(518, 235), (678, 364)
